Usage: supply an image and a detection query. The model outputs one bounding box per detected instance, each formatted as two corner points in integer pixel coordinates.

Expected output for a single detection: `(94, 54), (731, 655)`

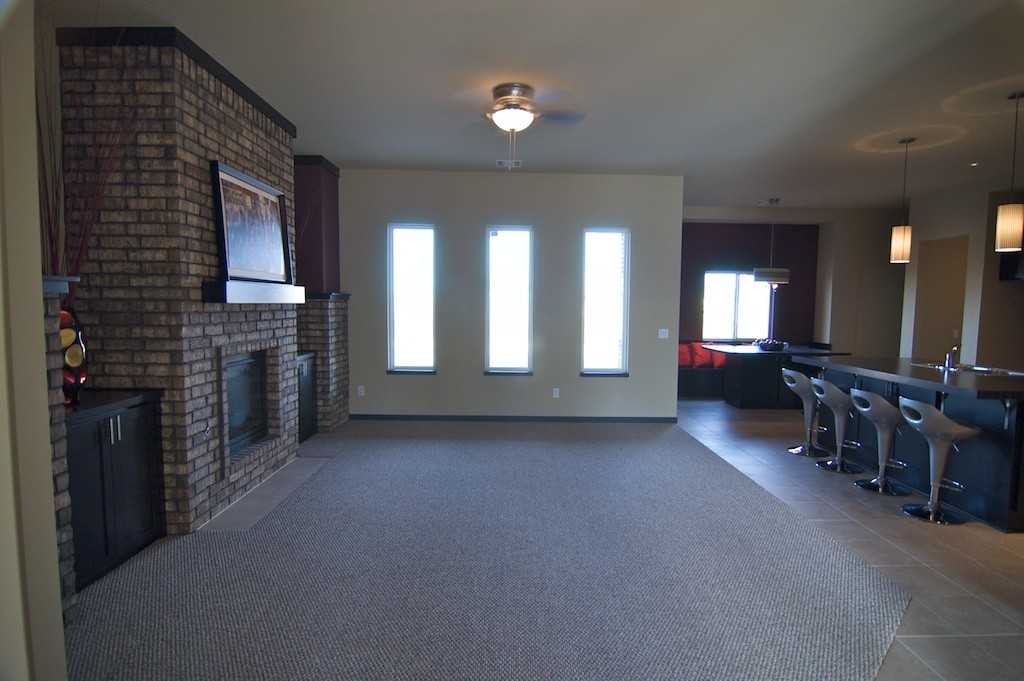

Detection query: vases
(59, 306), (89, 404)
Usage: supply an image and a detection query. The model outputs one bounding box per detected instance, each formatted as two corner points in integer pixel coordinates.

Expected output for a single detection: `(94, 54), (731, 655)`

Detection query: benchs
(679, 339), (754, 397)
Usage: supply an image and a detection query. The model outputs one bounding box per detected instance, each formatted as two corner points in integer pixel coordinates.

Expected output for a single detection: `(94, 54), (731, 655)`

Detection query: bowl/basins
(760, 343), (784, 351)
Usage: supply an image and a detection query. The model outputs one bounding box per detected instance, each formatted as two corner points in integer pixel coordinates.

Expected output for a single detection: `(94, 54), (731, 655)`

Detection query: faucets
(936, 344), (962, 372)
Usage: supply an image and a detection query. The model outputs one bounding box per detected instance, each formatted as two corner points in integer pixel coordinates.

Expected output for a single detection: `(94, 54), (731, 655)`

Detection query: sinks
(910, 363), (1024, 377)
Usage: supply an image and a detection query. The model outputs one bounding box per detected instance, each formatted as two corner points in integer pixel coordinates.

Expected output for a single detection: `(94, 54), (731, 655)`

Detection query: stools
(810, 377), (865, 473)
(896, 397), (981, 526)
(782, 368), (831, 458)
(848, 388), (913, 495)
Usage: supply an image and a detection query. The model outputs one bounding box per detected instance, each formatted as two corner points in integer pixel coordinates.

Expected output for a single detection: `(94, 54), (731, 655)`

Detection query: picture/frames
(209, 158), (295, 285)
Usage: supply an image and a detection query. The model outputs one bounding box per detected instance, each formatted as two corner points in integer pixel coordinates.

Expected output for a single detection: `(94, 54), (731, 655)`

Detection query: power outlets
(553, 388), (559, 397)
(358, 386), (365, 395)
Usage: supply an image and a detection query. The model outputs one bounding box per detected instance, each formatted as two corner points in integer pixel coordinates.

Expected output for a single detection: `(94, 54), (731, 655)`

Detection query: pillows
(679, 342), (751, 368)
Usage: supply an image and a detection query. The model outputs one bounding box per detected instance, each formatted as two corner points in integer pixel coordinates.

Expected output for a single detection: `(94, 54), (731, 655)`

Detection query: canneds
(945, 353), (952, 367)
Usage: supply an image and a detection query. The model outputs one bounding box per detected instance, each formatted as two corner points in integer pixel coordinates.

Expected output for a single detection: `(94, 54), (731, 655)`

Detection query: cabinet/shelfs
(297, 351), (318, 442)
(67, 401), (167, 586)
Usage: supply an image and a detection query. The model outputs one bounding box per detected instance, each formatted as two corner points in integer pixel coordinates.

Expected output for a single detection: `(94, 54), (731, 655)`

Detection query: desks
(700, 345), (852, 411)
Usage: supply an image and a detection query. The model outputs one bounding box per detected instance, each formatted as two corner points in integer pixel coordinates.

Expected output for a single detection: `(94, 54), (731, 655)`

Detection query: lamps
(754, 198), (791, 284)
(487, 81), (536, 135)
(889, 137), (915, 264)
(995, 89), (1024, 253)
